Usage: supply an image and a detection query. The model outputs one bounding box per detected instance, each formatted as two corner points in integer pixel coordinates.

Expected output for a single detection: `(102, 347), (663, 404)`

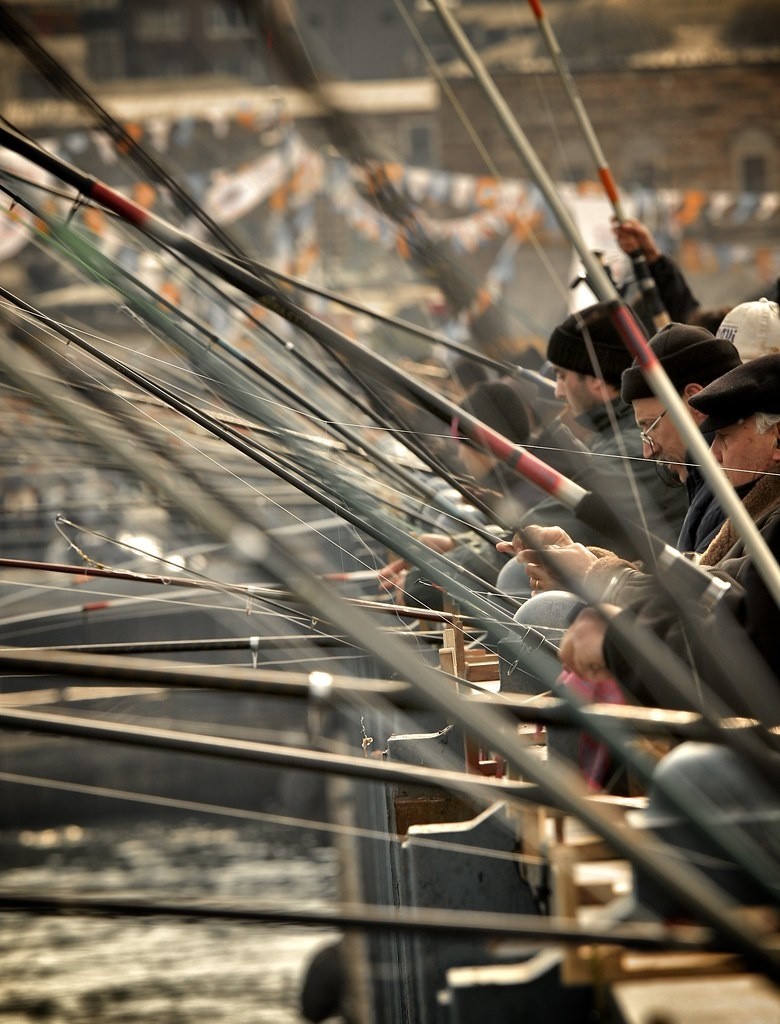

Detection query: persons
(381, 216), (780, 927)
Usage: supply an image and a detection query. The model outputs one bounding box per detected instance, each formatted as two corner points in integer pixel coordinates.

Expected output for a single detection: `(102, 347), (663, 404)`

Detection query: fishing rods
(2, 0), (780, 990)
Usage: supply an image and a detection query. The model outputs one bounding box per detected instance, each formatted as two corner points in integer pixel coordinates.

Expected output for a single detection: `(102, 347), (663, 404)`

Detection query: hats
(687, 352), (780, 433)
(547, 301), (648, 385)
(717, 298), (780, 364)
(455, 384), (529, 451)
(621, 322), (742, 405)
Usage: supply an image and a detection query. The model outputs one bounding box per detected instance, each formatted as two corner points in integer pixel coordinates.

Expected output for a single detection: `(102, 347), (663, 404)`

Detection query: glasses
(640, 409), (669, 447)
